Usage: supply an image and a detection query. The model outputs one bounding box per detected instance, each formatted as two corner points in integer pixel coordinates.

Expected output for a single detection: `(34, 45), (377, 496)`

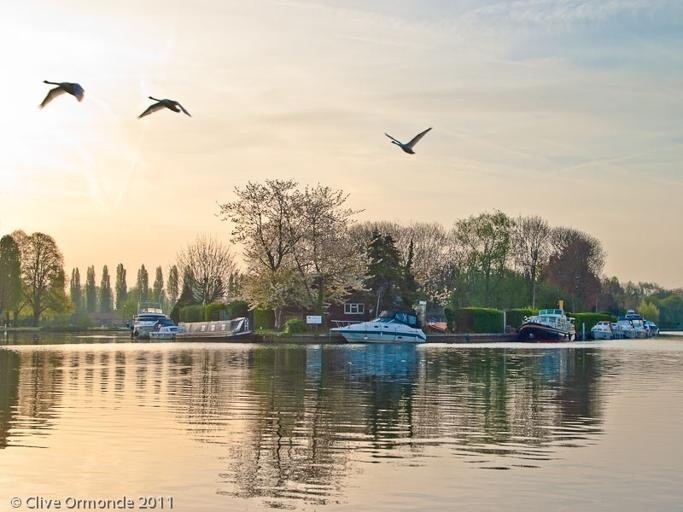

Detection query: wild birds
(138, 95), (192, 119)
(382, 127), (433, 156)
(39, 79), (86, 111)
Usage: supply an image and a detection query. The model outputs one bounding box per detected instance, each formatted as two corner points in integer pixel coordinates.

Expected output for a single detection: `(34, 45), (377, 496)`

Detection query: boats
(329, 310), (426, 344)
(518, 308), (575, 343)
(126, 307), (252, 340)
(590, 319), (659, 340)
